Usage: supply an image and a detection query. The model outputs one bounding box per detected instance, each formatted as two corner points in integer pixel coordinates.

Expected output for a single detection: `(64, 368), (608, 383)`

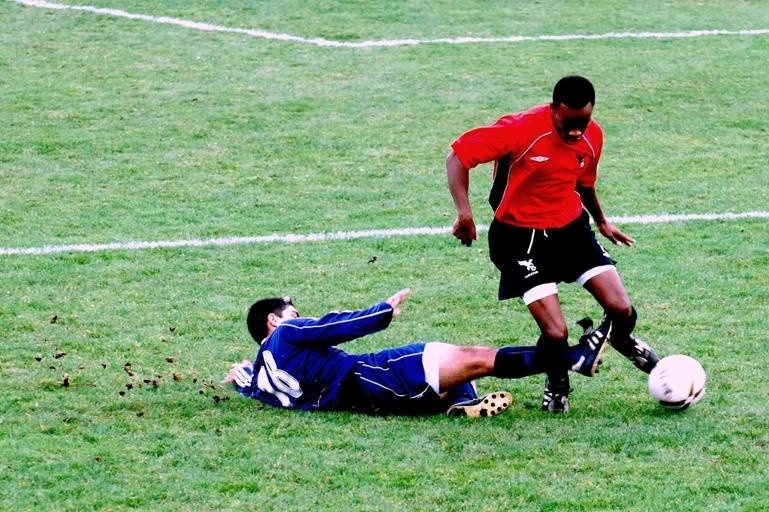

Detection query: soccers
(648, 354), (706, 410)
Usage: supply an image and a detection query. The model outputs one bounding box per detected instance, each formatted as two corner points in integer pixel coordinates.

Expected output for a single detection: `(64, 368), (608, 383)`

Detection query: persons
(447, 77), (660, 414)
(225, 287), (613, 417)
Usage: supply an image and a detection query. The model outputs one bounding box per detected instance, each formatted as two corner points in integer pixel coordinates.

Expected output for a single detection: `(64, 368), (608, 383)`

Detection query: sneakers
(447, 391), (513, 418)
(606, 331), (659, 374)
(576, 315), (613, 377)
(541, 376), (569, 414)
